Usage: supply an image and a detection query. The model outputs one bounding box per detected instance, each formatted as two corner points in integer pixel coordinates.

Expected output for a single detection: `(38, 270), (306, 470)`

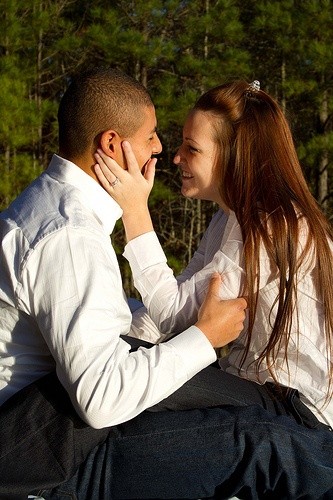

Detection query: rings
(112, 178), (118, 185)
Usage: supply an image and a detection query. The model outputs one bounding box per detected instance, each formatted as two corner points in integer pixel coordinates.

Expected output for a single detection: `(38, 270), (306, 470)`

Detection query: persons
(0, 66), (333, 500)
(1, 77), (331, 500)
